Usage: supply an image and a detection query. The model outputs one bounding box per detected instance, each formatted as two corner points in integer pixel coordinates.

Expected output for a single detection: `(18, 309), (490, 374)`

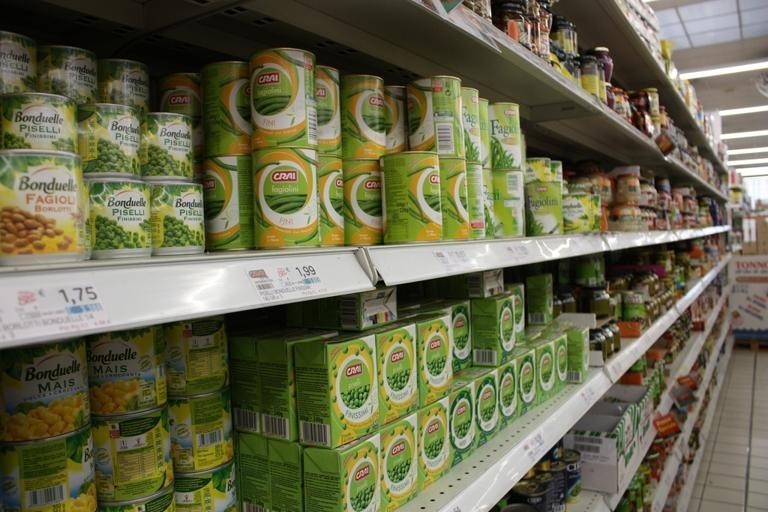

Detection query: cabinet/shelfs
(0, 2), (740, 512)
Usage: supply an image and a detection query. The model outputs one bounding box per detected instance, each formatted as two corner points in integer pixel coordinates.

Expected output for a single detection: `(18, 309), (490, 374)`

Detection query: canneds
(461, 0), (697, 174)
(0, 28), (697, 280)
(0, 243), (698, 511)
